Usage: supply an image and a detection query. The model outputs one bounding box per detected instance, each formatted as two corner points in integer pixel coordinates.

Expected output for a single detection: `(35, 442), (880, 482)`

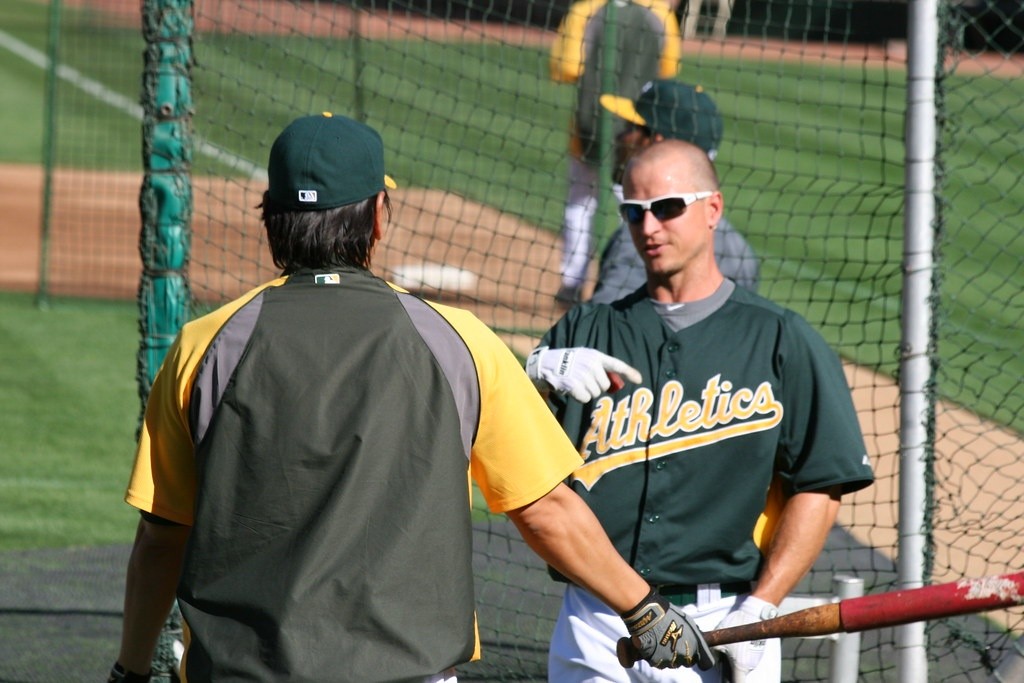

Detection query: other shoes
(554, 288), (579, 308)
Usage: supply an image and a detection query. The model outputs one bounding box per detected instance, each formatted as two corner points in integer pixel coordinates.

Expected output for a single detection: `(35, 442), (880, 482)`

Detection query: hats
(600, 77), (723, 156)
(267, 113), (398, 211)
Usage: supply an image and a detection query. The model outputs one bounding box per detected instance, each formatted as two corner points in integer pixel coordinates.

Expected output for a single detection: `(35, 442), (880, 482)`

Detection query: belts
(655, 579), (752, 606)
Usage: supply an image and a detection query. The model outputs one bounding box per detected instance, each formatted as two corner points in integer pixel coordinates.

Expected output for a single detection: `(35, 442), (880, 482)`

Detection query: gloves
(526, 344), (643, 405)
(621, 585), (717, 672)
(711, 595), (782, 683)
(106, 660), (152, 683)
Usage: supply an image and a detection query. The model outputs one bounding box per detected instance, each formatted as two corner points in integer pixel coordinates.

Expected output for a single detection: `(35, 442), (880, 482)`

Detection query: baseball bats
(615, 569), (1023, 671)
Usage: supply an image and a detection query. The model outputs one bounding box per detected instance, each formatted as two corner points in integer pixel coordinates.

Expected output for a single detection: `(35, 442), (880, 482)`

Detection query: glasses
(619, 192), (717, 224)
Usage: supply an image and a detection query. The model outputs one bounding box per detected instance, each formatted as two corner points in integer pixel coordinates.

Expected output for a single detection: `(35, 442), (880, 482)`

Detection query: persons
(550, 1), (683, 305)
(590, 77), (759, 304)
(526, 139), (873, 683)
(110, 112), (715, 683)
